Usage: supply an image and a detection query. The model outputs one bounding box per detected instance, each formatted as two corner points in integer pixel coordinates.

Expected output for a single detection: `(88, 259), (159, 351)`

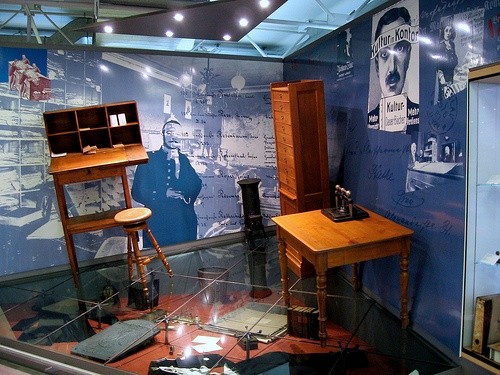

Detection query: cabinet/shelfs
(43, 100), (142, 156)
(270, 80), (331, 280)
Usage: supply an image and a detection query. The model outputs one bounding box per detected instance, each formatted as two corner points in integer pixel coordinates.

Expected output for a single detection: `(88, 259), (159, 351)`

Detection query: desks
(47, 145), (149, 288)
(270, 204), (414, 348)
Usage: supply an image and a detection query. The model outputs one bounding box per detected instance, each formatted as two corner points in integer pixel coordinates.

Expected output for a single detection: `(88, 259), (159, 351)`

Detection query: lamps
(177, 58), (245, 115)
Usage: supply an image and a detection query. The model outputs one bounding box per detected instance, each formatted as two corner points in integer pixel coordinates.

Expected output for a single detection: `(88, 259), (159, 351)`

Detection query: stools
(114, 206), (176, 309)
